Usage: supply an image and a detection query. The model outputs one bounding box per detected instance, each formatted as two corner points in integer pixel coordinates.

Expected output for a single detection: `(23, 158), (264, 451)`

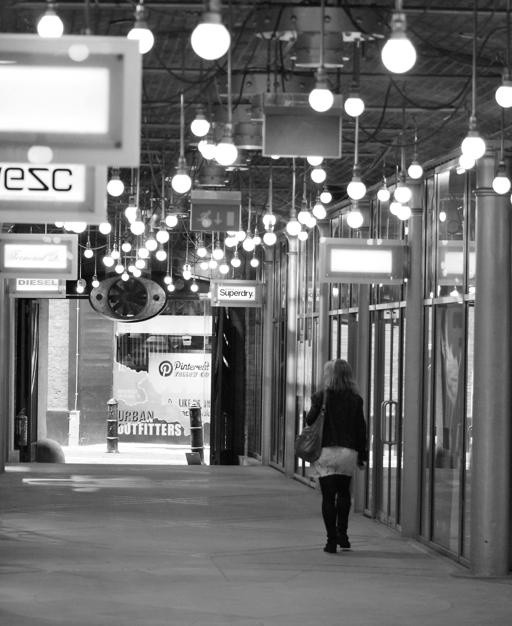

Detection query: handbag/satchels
(295, 413), (326, 465)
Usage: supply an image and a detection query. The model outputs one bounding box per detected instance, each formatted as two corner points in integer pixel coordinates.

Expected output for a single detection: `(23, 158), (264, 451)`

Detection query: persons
(306, 359), (368, 553)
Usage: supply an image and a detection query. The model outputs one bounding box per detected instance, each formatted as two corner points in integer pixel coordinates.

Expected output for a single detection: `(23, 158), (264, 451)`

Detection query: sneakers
(324, 540), (337, 554)
(337, 530), (351, 549)
